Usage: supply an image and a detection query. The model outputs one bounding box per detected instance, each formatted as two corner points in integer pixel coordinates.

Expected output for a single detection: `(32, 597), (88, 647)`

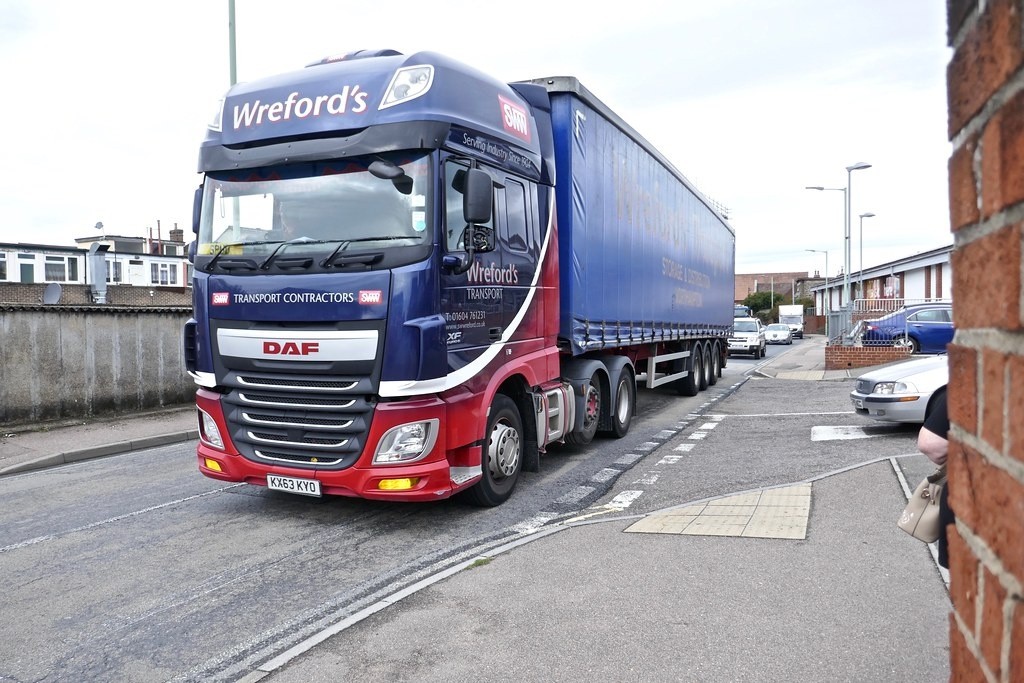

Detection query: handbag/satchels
(898, 461), (949, 543)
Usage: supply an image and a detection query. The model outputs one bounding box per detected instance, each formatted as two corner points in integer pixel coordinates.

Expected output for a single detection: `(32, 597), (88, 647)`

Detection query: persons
(916, 383), (956, 570)
(275, 200), (309, 241)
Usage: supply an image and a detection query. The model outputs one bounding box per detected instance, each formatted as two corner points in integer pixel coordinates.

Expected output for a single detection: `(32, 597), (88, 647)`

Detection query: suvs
(726, 317), (766, 359)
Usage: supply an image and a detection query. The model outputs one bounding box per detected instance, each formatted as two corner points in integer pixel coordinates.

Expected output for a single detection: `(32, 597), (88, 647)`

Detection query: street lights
(859, 213), (876, 299)
(805, 250), (827, 314)
(806, 186), (847, 302)
(846, 162), (872, 306)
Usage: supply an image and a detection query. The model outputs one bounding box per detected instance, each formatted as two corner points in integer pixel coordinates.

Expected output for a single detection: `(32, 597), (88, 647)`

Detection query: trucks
(779, 305), (806, 339)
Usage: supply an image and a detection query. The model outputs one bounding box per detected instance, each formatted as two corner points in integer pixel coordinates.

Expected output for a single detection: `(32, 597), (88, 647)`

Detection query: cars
(860, 303), (955, 354)
(850, 350), (950, 423)
(765, 324), (792, 345)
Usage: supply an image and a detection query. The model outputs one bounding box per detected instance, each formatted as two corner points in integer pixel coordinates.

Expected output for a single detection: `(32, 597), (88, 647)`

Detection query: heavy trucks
(183, 49), (735, 509)
